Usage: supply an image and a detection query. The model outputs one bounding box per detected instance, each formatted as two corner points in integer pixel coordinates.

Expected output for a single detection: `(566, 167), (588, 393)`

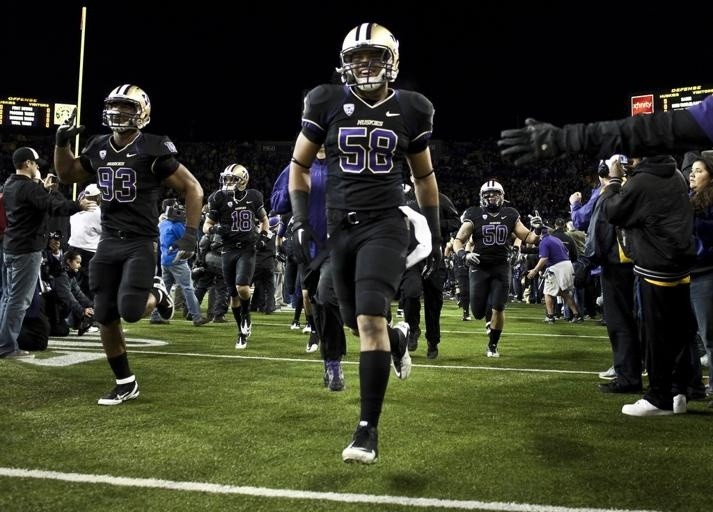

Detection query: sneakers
(97, 374), (140, 406)
(395, 309), (404, 318)
(426, 339), (438, 359)
(597, 352), (712, 417)
(289, 318), (345, 391)
(392, 321), (412, 380)
(151, 275), (175, 321)
(544, 314), (591, 323)
(340, 419), (378, 464)
(408, 327), (420, 351)
(149, 307), (251, 351)
(462, 317), (499, 358)
(3, 348), (34, 358)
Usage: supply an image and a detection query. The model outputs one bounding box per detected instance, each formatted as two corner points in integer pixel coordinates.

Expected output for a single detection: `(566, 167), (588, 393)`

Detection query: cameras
(620, 155), (630, 165)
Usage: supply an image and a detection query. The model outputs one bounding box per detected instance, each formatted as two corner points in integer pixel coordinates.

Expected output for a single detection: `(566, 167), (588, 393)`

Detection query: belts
(234, 242), (241, 248)
(113, 230), (128, 240)
(328, 211), (386, 225)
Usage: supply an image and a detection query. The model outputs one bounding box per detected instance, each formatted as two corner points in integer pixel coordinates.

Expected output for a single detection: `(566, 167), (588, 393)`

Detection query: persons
(494, 90), (713, 171)
(395, 151), (712, 416)
(283, 19), (441, 467)
(50, 81), (203, 409)
(150, 163), (320, 354)
(0, 147), (100, 358)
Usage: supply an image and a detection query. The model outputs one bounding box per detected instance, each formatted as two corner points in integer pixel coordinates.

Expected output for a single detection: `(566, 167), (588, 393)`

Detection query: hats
(83, 183), (101, 197)
(12, 146), (39, 163)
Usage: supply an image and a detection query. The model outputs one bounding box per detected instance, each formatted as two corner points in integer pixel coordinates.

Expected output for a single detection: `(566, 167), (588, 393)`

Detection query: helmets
(101, 83), (151, 133)
(478, 180), (505, 212)
(335, 22), (400, 93)
(218, 162), (249, 197)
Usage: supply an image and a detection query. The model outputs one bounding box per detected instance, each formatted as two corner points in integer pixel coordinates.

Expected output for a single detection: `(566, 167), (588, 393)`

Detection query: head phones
(598, 158), (609, 178)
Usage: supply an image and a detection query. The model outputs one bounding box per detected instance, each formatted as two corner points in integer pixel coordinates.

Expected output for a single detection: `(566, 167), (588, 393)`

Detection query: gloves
(57, 107), (86, 140)
(212, 224), (230, 235)
(287, 218), (324, 265)
(421, 238), (444, 280)
(274, 249), (286, 262)
(460, 251), (481, 268)
(255, 235), (271, 250)
(573, 263), (592, 290)
(528, 210), (543, 229)
(497, 118), (564, 168)
(167, 238), (196, 264)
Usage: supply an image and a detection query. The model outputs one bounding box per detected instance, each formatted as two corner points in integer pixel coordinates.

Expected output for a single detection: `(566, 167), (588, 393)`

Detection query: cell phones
(52, 176), (58, 183)
